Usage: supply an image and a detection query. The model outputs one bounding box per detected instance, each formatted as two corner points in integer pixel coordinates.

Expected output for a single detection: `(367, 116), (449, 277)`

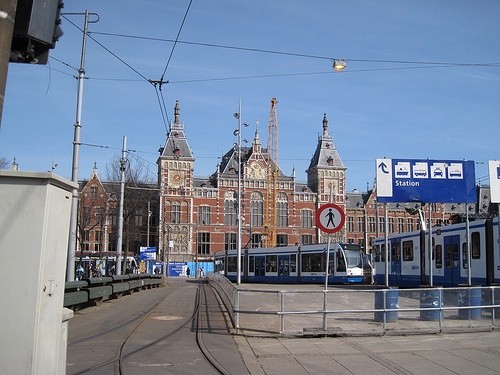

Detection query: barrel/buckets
(457, 283), (482, 320)
(419, 286), (444, 322)
(374, 286), (399, 323)
(490, 283), (500, 318)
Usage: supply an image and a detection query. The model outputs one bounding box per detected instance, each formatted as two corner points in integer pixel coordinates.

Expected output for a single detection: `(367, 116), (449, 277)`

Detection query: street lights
(147, 201), (153, 247)
(233, 98), (250, 328)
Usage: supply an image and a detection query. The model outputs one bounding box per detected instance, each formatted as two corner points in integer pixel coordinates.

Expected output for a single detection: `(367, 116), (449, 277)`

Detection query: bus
(372, 215), (500, 288)
(214, 242), (365, 284)
(74, 256), (140, 278)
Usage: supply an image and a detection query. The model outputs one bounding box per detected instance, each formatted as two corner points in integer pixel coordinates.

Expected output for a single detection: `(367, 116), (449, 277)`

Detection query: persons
(154, 267), (160, 275)
(93, 267), (98, 277)
(199, 267), (204, 278)
(76, 265), (84, 279)
(187, 268), (190, 278)
(109, 268), (114, 275)
(126, 266), (133, 274)
